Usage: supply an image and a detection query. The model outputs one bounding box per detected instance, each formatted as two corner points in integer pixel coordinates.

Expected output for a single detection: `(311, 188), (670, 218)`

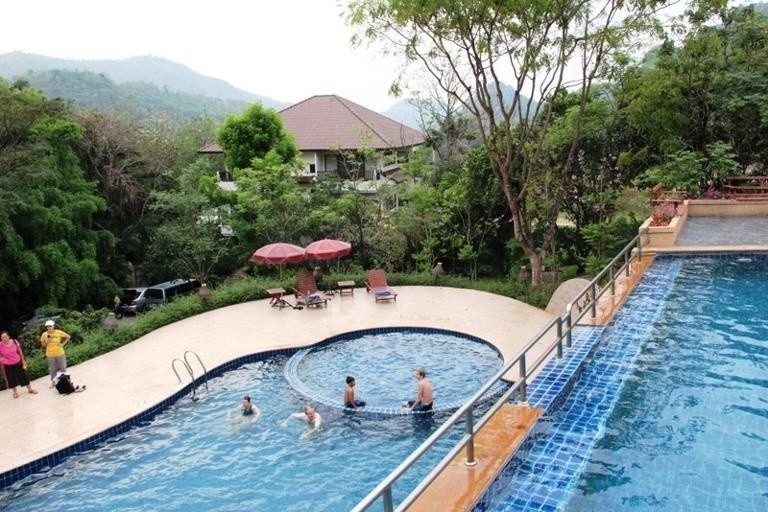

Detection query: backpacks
(56, 374), (76, 394)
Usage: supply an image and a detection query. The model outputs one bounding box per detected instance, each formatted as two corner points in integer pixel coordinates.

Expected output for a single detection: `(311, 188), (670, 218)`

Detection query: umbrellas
(301, 238), (352, 293)
(251, 242), (305, 299)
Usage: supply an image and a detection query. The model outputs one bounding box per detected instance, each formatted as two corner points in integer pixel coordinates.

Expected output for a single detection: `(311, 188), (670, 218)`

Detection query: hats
(44, 320), (55, 327)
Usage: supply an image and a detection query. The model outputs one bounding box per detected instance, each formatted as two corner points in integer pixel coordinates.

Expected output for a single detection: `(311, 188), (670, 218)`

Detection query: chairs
(363, 270), (398, 303)
(296, 273), (331, 310)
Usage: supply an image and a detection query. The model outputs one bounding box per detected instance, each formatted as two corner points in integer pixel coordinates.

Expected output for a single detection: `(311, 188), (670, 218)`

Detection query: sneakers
(29, 389), (37, 394)
(13, 393), (19, 398)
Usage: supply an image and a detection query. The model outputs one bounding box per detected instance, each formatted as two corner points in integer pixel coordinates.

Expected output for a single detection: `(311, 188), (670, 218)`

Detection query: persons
(40, 320), (71, 389)
(0, 331), (38, 398)
(227, 396), (260, 422)
(285, 405), (322, 434)
(344, 376), (366, 409)
(407, 368), (434, 411)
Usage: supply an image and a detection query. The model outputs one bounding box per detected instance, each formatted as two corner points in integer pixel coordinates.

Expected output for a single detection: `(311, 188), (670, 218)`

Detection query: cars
(117, 285), (149, 316)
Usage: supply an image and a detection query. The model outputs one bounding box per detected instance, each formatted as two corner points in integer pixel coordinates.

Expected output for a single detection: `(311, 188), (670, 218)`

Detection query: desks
(266, 287), (286, 307)
(337, 280), (355, 297)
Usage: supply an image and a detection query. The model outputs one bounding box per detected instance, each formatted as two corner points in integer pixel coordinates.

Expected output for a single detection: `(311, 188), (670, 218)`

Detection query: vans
(144, 276), (203, 311)
